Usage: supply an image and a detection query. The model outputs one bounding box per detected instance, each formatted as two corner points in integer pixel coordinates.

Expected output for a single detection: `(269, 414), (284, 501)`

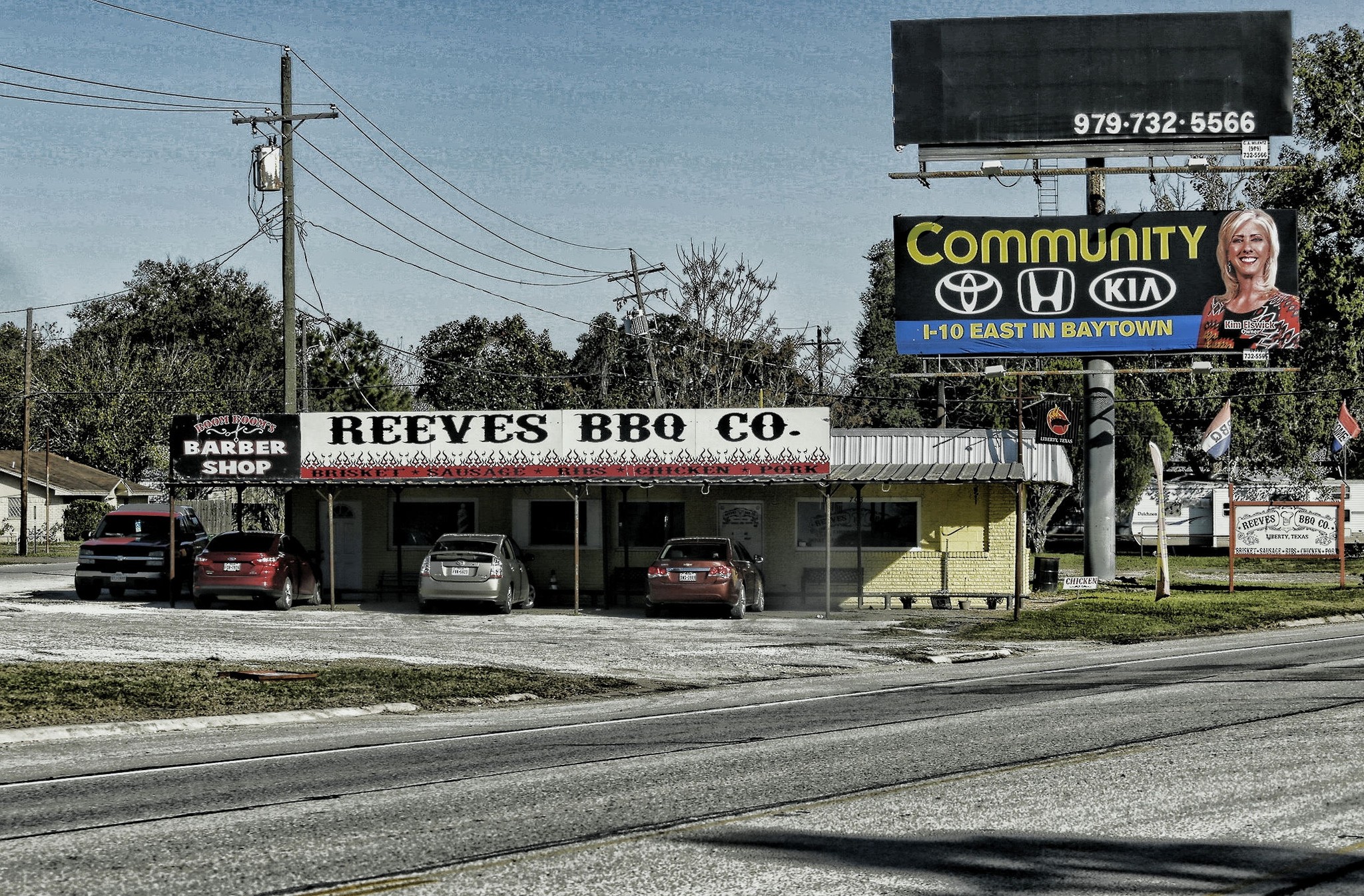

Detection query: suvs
(73, 503), (206, 603)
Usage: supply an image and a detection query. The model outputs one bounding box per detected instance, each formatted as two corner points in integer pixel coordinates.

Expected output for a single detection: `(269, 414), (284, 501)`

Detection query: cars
(193, 529), (326, 610)
(644, 538), (766, 620)
(417, 534), (540, 615)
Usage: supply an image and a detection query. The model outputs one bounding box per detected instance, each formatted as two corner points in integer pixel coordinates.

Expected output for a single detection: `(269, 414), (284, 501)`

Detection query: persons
(1197, 209), (1300, 349)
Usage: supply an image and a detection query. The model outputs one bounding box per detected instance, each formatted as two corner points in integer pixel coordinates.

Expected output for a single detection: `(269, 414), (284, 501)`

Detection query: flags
(1147, 441), (1171, 602)
(1200, 398), (1232, 460)
(1329, 397), (1362, 455)
